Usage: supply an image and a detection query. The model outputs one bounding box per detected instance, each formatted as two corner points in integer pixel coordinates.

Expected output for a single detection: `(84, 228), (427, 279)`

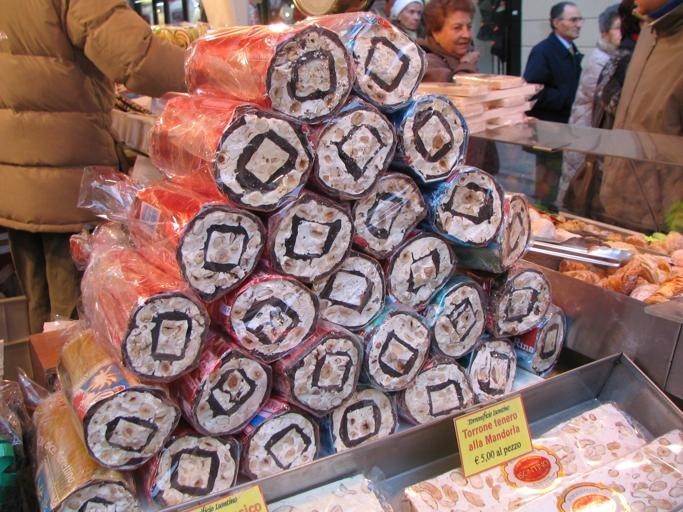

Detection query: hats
(384, 0), (424, 20)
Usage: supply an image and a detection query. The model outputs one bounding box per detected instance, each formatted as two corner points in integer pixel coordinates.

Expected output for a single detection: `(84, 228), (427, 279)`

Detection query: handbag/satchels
(563, 158), (597, 216)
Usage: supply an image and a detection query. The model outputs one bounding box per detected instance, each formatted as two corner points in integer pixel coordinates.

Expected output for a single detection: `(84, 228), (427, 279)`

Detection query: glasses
(558, 17), (582, 23)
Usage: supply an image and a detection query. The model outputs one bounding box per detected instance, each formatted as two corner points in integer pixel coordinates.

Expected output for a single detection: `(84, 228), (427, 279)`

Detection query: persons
(382, 0), (426, 46)
(554, 3), (625, 212)
(567, 1), (683, 234)
(588, 1), (649, 218)
(0, 0), (191, 337)
(407, 1), (482, 84)
(521, 1), (585, 210)
